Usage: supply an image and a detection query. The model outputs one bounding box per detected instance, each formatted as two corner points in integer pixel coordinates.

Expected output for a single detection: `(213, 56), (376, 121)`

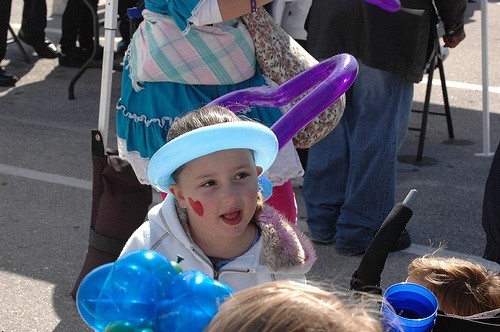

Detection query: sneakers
(310, 225), (411, 256)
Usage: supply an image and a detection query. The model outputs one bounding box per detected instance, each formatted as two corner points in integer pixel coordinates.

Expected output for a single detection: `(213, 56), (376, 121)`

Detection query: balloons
(76, 249), (235, 332)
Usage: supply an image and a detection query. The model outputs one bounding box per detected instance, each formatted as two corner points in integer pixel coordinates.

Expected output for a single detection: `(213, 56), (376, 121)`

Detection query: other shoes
(57, 39), (128, 68)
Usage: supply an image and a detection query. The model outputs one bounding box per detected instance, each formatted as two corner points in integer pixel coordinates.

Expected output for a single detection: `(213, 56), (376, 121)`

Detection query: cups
(380, 282), (439, 332)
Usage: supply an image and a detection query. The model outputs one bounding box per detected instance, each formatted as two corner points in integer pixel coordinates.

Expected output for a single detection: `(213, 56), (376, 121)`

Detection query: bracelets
(250, 0), (256, 13)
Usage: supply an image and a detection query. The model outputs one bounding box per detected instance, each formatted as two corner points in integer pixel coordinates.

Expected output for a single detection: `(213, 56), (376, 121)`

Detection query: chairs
(408, 2), (454, 162)
(68, 0), (124, 100)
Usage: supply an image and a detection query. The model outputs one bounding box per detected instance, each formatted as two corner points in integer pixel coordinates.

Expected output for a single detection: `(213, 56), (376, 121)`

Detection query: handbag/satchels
(239, 6), (347, 150)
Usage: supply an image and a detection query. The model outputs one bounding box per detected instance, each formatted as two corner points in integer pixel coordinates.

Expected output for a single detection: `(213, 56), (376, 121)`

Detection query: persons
(206, 277), (405, 332)
(305, 1), (468, 257)
(0, 0), (58, 87)
(106, 0), (304, 226)
(96, 106), (318, 332)
(60, 0), (118, 65)
(405, 257), (500, 318)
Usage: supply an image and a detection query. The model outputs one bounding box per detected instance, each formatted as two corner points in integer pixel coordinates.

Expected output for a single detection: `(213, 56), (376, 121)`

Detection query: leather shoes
(0, 66), (18, 86)
(18, 29), (60, 58)
